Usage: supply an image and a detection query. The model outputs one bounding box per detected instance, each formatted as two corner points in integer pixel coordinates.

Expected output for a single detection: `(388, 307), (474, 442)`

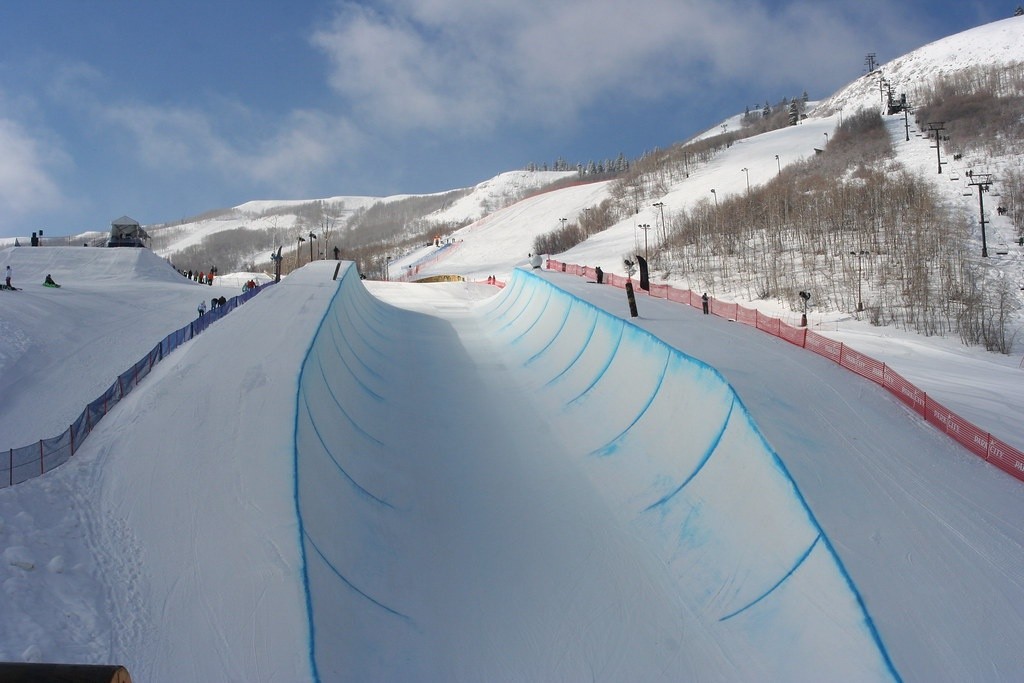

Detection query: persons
(210, 296), (227, 310)
(333, 246), (340, 260)
(242, 279), (255, 292)
(5, 265), (12, 288)
(200, 272), (203, 283)
(197, 300), (207, 317)
(188, 269), (192, 280)
(45, 274), (56, 285)
(210, 265), (217, 275)
(207, 273), (213, 283)
(193, 269), (199, 281)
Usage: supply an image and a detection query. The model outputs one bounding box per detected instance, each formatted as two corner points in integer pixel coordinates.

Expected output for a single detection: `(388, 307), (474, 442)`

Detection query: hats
(48, 274), (51, 276)
